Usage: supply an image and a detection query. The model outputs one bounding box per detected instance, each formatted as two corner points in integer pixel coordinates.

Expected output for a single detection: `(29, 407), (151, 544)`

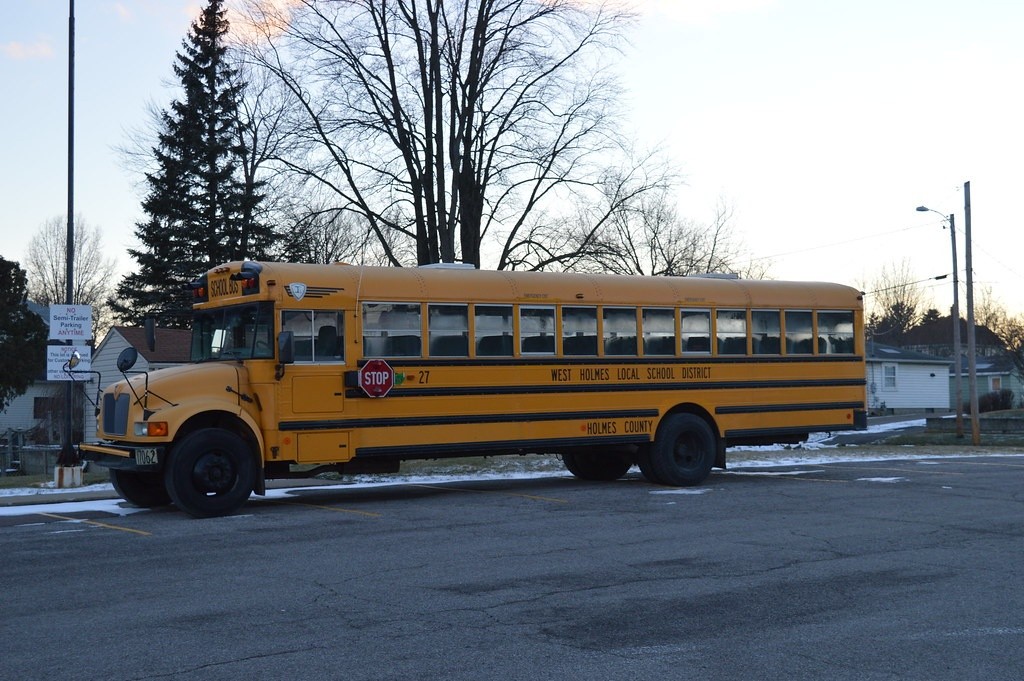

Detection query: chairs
(382, 334), (849, 356)
(316, 325), (337, 355)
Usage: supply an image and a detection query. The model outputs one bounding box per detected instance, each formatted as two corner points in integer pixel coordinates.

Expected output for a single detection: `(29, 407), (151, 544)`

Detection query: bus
(61, 257), (869, 517)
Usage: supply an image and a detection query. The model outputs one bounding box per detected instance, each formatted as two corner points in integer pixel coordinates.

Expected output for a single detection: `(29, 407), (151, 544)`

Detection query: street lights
(918, 202), (968, 437)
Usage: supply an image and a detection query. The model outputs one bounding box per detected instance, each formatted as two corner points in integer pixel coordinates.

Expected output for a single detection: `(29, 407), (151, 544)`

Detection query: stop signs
(358, 358), (395, 398)
